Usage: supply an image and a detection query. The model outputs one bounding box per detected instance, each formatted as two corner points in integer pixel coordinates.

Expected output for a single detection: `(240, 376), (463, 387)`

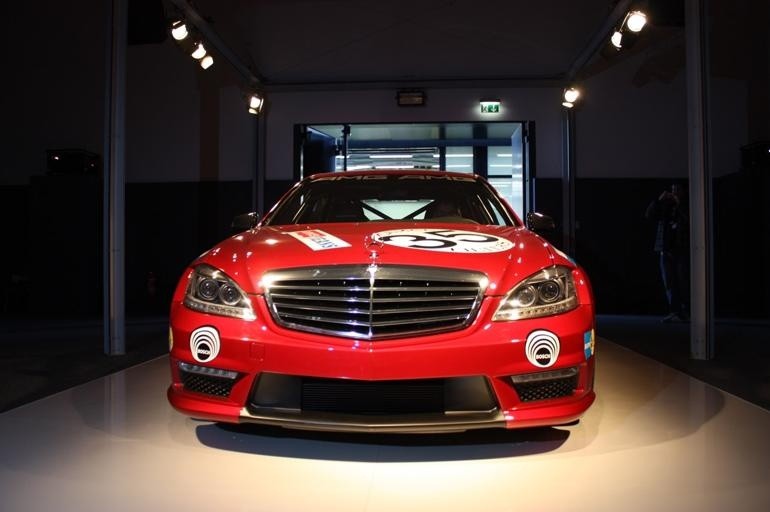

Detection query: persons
(645, 183), (688, 334)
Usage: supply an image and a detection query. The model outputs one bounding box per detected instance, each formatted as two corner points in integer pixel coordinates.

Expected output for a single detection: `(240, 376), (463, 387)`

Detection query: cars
(167, 170), (596, 436)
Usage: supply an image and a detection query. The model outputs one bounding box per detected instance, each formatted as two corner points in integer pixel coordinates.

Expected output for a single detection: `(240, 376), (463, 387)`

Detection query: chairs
(318, 197), (369, 221)
(424, 197), (465, 219)
